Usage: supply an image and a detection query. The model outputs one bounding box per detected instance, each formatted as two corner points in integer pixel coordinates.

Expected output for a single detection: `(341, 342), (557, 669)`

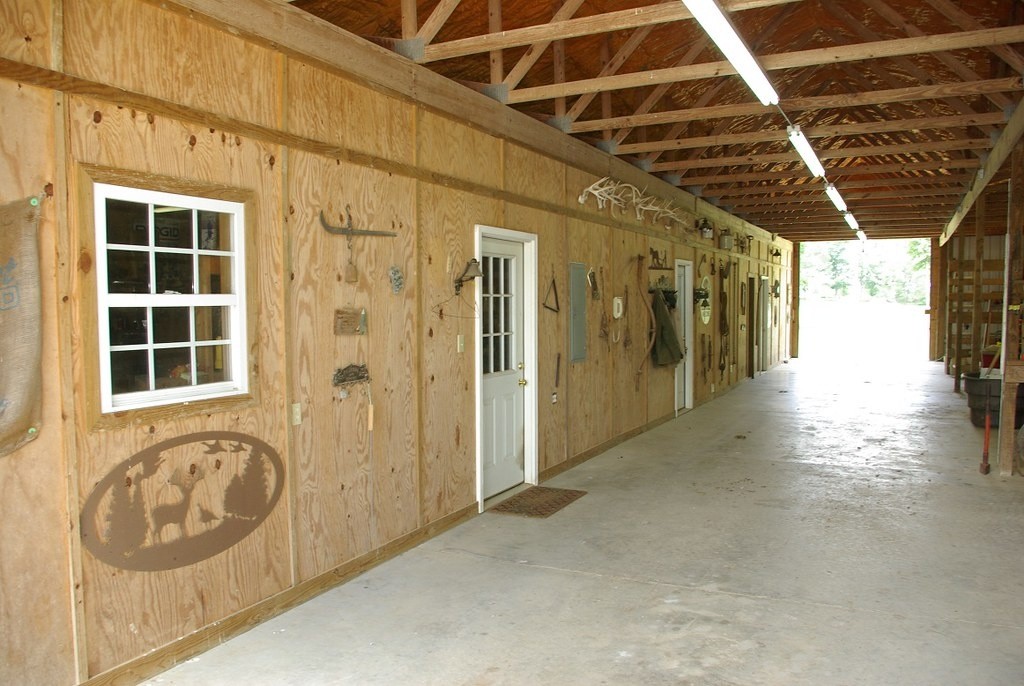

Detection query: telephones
(613, 297), (624, 320)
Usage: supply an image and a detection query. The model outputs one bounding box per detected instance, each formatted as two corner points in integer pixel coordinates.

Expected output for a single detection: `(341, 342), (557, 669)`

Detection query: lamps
(823, 182), (848, 212)
(692, 289), (710, 314)
(770, 248), (782, 259)
(694, 217), (712, 233)
(786, 124), (827, 177)
(454, 258), (485, 290)
(682, 0), (780, 106)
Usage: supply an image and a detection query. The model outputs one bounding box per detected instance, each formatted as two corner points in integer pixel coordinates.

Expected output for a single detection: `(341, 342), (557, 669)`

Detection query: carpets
(483, 484), (588, 520)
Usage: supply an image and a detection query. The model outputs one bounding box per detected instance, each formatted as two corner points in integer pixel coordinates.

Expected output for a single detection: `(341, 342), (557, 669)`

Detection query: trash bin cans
(961, 372), (1024, 430)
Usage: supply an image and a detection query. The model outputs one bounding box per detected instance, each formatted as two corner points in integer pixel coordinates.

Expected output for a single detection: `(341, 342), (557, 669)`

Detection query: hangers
(430, 291), (483, 319)
